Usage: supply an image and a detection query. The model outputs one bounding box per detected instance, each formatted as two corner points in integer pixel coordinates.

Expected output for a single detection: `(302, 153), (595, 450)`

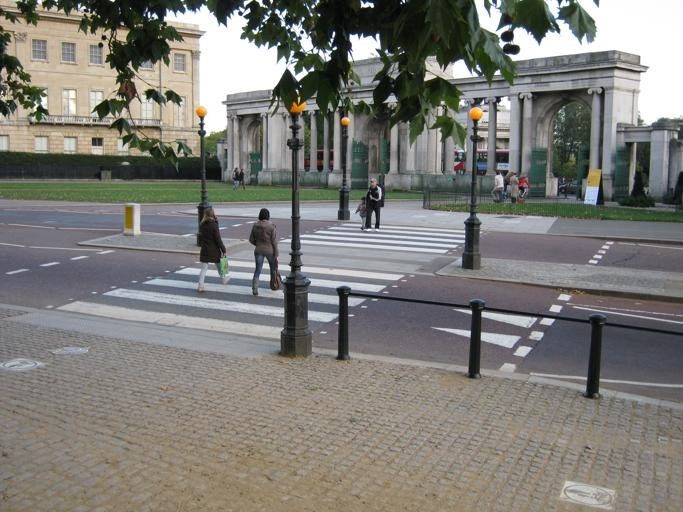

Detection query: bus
(452, 148), (509, 176)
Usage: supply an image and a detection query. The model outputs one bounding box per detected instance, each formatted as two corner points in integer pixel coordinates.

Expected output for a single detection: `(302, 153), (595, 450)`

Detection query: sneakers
(223, 276), (230, 284)
(197, 288), (207, 292)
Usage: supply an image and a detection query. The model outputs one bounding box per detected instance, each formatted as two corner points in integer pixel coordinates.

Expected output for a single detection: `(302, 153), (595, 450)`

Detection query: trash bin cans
(100, 166), (112, 183)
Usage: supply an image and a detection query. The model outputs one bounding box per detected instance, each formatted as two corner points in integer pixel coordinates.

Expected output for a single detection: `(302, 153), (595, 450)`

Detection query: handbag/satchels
(219, 256), (229, 275)
(270, 260), (282, 290)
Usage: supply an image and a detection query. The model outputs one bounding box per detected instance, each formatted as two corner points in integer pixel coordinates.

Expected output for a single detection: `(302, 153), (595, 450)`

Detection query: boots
(253, 278), (259, 295)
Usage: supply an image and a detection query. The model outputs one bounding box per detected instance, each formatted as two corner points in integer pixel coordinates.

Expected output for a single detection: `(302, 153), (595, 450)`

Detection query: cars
(560, 178), (583, 195)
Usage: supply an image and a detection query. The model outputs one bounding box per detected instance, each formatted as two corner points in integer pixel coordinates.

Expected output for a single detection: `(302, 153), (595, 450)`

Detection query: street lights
(195, 106), (211, 250)
(337, 116), (353, 222)
(278, 95), (313, 361)
(460, 106), (483, 272)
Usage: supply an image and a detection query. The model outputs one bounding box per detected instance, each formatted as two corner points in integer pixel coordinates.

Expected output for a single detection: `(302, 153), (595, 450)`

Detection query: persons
(490, 169), (531, 204)
(249, 208), (284, 296)
(365, 178), (383, 233)
(355, 196), (369, 232)
(231, 168), (245, 191)
(196, 208), (232, 294)
